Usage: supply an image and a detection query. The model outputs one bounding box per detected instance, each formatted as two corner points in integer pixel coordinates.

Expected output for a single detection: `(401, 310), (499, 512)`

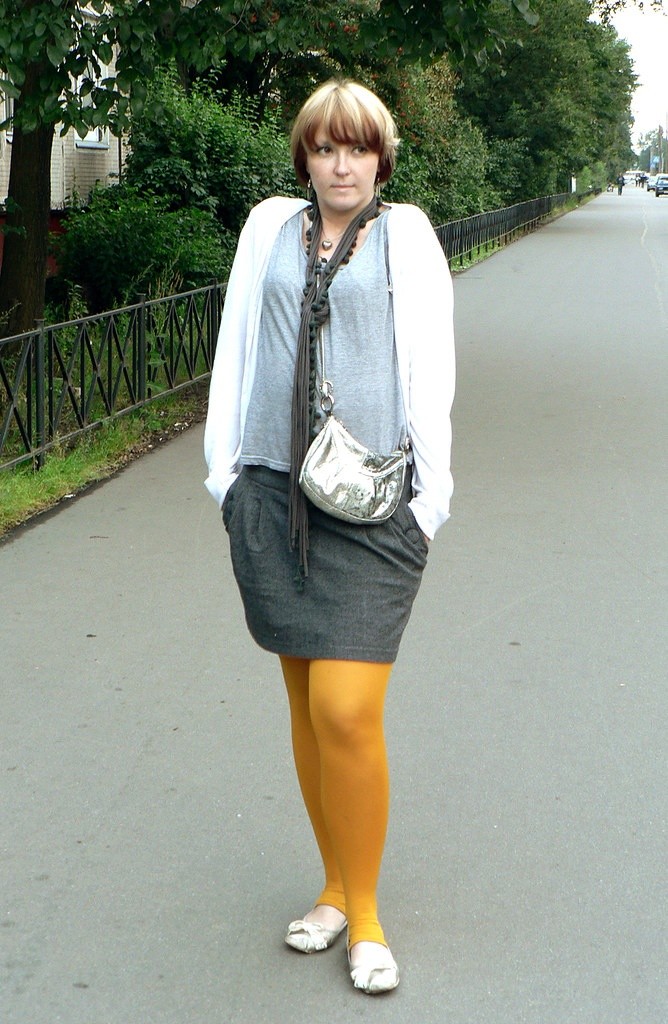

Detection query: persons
(635, 173), (649, 188)
(607, 172), (624, 196)
(205, 77), (455, 994)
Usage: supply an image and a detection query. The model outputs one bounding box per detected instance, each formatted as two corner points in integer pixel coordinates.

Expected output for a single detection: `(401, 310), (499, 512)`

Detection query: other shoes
(284, 904), (348, 953)
(345, 924), (400, 994)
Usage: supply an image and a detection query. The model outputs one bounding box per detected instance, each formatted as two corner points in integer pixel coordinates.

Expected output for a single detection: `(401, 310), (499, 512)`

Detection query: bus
(623, 171), (645, 180)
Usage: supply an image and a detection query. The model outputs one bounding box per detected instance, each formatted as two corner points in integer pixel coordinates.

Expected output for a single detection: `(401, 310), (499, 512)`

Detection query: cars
(646, 177), (655, 191)
(655, 175), (668, 197)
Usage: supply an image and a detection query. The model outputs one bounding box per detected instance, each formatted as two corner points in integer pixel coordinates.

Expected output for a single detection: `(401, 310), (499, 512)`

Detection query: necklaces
(321, 225), (347, 251)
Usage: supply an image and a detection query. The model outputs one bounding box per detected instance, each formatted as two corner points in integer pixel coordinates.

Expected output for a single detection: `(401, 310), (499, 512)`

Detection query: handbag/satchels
(298, 416), (408, 524)
